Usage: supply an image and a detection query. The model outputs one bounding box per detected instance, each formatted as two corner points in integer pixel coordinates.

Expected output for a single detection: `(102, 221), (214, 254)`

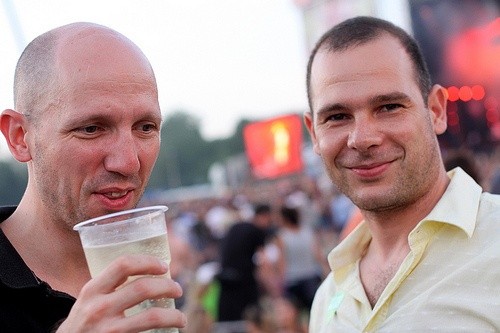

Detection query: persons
(164, 154), (498, 333)
(304, 15), (500, 333)
(0, 21), (188, 333)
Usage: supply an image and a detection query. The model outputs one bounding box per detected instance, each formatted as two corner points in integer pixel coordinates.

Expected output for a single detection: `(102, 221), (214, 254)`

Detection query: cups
(72, 205), (180, 333)
